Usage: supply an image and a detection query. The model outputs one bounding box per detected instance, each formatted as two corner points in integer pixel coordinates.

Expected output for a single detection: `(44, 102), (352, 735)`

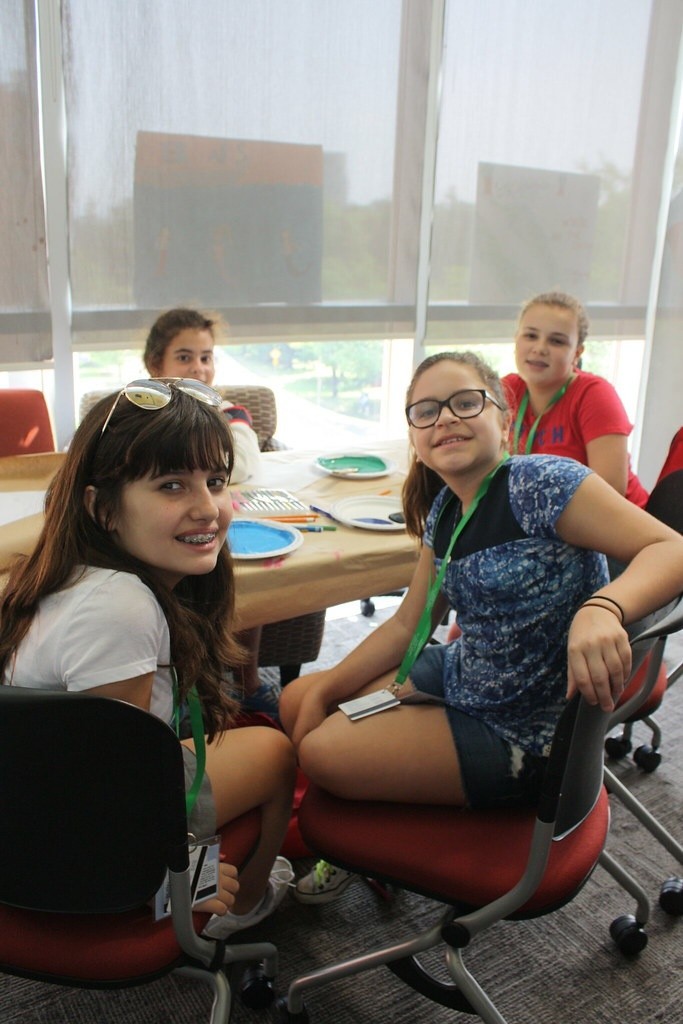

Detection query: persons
(279, 351), (683, 904)
(142, 309), (285, 717)
(0, 383), (296, 943)
(501, 292), (683, 536)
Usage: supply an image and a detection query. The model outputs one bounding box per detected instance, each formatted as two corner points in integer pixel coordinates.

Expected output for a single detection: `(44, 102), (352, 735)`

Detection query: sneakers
(229, 678), (285, 720)
(294, 859), (356, 904)
(199, 855), (296, 941)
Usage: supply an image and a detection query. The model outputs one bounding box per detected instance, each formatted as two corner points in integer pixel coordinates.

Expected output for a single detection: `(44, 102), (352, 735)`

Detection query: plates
(224, 516), (304, 560)
(312, 450), (399, 480)
(332, 494), (408, 531)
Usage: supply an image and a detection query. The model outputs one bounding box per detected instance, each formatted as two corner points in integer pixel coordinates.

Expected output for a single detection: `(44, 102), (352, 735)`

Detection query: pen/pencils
(228, 488), (307, 513)
(332, 467), (359, 474)
(310, 504), (335, 520)
(258, 513), (336, 532)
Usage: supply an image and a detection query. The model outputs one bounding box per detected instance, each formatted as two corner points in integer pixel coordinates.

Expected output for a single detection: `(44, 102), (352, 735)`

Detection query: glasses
(405, 390), (505, 429)
(97, 376), (223, 444)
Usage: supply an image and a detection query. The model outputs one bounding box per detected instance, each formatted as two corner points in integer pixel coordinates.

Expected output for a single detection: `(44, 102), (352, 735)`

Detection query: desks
(0, 436), (430, 717)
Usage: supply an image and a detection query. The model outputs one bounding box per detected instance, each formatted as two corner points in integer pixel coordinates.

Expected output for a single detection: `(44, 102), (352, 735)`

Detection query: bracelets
(579, 596), (623, 624)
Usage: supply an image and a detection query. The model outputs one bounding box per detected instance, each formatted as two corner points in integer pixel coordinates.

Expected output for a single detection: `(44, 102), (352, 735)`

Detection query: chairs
(0, 384), (683, 1020)
